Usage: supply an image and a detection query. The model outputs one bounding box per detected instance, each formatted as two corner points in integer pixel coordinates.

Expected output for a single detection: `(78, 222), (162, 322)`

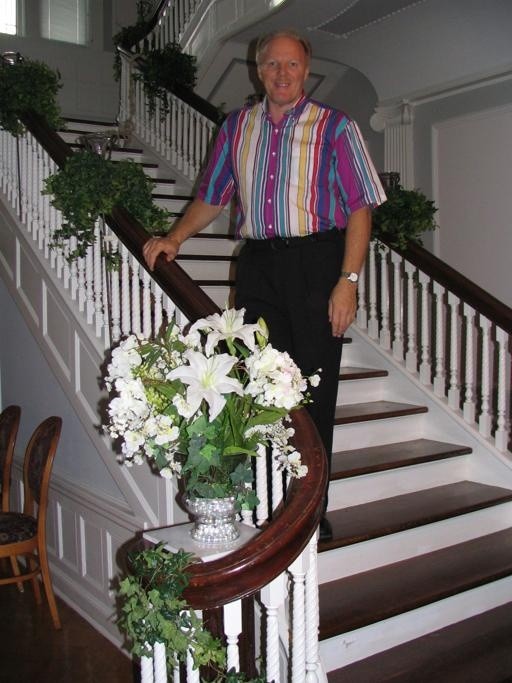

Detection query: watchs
(340, 269), (360, 282)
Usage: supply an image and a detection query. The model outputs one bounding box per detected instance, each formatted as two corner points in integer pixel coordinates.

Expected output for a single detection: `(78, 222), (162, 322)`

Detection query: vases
(179, 492), (241, 542)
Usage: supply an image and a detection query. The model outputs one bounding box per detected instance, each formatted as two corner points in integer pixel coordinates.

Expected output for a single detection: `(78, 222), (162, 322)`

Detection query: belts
(248, 228), (335, 250)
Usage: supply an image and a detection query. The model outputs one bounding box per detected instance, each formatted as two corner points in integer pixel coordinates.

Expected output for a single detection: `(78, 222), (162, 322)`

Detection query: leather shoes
(320, 517), (333, 540)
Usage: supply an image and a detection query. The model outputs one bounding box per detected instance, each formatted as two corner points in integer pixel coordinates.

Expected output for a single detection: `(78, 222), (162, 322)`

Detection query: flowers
(99, 302), (324, 509)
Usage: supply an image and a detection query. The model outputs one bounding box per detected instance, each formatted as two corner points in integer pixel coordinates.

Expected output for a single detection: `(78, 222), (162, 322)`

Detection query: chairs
(0, 404), (64, 631)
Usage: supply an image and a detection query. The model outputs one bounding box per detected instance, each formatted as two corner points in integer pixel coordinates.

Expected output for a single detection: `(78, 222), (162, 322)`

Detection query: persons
(140, 28), (390, 542)
